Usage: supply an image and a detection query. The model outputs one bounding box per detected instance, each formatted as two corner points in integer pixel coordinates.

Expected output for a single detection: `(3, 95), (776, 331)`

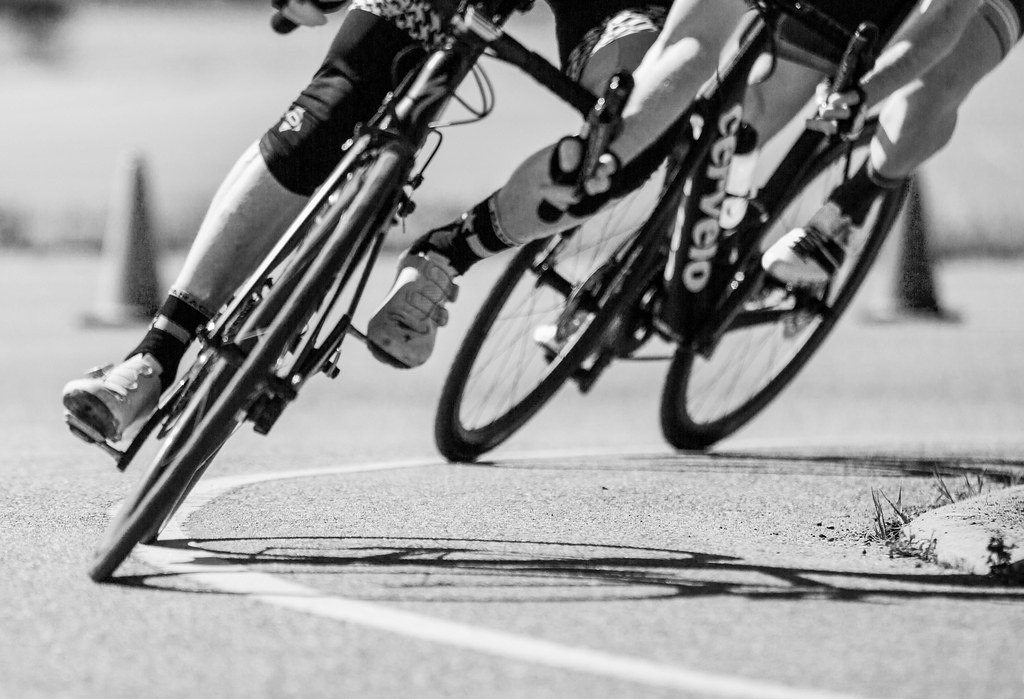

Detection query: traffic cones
(60, 154), (164, 329)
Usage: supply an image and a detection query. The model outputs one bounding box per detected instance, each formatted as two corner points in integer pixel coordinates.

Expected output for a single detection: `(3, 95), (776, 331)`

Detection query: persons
(535, 0), (1024, 357)
(61, 0), (747, 443)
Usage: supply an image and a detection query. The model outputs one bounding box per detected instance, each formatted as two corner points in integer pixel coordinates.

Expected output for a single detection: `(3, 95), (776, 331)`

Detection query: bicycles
(435, 0), (910, 463)
(86, 0), (636, 583)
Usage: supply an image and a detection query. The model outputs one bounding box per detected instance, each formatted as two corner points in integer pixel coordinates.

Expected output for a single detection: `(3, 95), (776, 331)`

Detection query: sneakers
(62, 352), (162, 443)
(532, 302), (589, 362)
(759, 199), (865, 287)
(366, 228), (459, 371)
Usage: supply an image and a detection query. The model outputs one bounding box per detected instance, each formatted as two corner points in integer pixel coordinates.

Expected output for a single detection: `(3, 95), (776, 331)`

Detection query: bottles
(717, 121), (760, 231)
